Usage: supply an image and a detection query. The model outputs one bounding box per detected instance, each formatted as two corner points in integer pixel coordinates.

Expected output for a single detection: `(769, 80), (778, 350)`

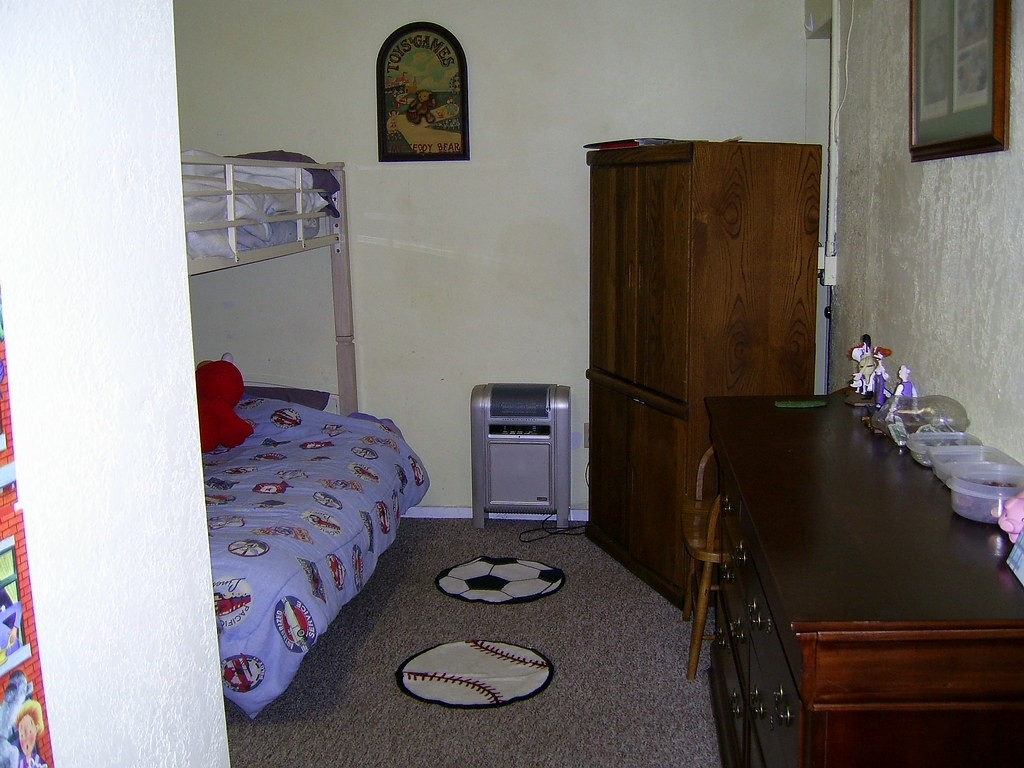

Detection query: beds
(179, 156), (430, 720)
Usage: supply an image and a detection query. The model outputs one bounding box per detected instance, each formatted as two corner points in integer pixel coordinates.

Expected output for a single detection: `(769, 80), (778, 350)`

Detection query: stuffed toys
(195, 353), (254, 452)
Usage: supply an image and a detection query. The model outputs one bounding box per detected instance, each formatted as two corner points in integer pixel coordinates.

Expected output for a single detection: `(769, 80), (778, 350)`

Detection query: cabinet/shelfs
(581, 139), (823, 609)
(702, 393), (1024, 768)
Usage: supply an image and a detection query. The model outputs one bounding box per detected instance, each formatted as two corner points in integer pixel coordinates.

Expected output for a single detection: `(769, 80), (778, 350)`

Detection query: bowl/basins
(928, 444), (1023, 486)
(945, 462), (1024, 524)
(907, 430), (984, 468)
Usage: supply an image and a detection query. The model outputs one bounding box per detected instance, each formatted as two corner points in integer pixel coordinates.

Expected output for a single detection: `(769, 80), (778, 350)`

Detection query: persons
(851, 334), (920, 421)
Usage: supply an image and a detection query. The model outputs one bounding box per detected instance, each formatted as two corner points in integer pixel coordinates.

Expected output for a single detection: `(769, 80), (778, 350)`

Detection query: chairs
(681, 444), (722, 680)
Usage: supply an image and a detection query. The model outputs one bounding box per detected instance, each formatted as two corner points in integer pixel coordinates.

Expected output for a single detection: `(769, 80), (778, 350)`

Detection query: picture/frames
(376, 21), (471, 163)
(909, 0), (1013, 164)
